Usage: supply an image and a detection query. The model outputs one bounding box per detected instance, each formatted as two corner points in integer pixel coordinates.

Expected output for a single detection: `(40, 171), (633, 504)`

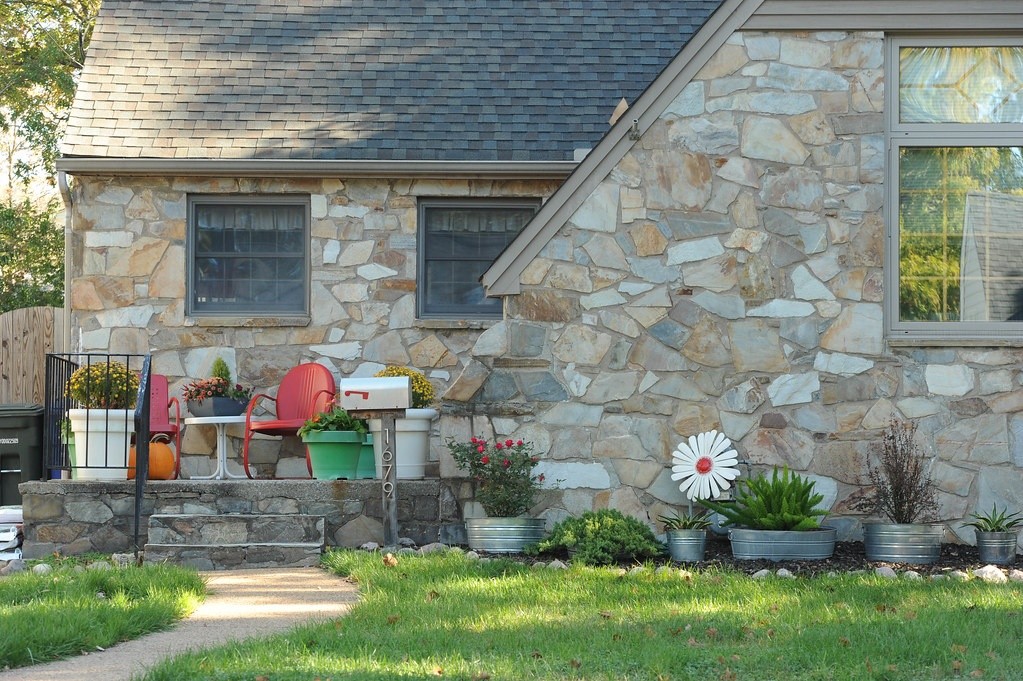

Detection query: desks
(184, 416), (255, 479)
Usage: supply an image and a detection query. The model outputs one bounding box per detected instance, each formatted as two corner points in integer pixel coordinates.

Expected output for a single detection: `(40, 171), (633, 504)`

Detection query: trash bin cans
(0, 402), (45, 506)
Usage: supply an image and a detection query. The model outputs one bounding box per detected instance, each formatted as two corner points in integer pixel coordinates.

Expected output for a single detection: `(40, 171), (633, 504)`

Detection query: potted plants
(958, 502), (1023, 566)
(694, 464), (837, 561)
(522, 508), (670, 565)
(297, 404), (367, 479)
(656, 508), (719, 562)
(842, 412), (944, 564)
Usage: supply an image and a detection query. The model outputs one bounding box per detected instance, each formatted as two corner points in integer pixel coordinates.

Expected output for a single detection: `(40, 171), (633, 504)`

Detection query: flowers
(671, 430), (741, 502)
(440, 433), (566, 517)
(374, 365), (433, 408)
(181, 357), (255, 403)
(63, 361), (138, 410)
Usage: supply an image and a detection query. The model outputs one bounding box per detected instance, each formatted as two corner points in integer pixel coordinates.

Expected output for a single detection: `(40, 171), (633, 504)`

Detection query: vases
(465, 517), (547, 556)
(356, 434), (376, 479)
(393, 409), (437, 481)
(68, 409), (135, 481)
(186, 396), (248, 417)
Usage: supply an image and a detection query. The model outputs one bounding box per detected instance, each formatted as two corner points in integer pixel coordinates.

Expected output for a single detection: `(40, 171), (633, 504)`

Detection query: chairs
(136, 374), (180, 480)
(245, 363), (335, 478)
